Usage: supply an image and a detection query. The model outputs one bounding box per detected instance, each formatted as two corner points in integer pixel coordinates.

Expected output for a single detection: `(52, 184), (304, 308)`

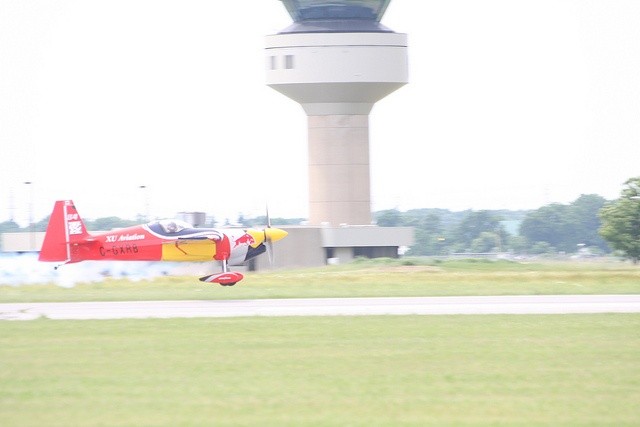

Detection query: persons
(166, 222), (176, 233)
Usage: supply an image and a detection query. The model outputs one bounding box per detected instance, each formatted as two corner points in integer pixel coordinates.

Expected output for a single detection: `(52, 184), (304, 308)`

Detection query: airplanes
(38, 201), (288, 286)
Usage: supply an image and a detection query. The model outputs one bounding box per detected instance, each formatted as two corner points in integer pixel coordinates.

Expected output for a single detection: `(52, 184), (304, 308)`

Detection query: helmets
(167, 223), (177, 231)
